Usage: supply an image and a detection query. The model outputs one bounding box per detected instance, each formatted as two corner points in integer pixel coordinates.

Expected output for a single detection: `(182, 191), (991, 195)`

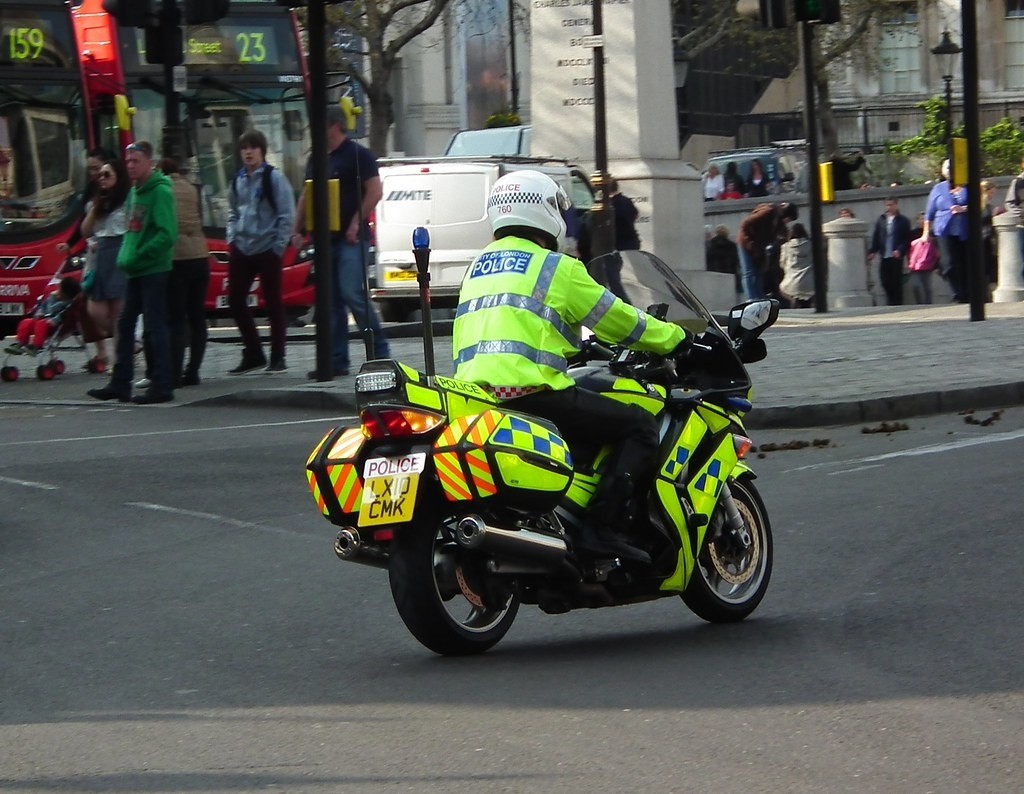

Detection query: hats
(782, 202), (798, 220)
(325, 104), (348, 128)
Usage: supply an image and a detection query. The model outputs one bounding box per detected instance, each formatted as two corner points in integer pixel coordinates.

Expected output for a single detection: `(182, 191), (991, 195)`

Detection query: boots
(575, 470), (652, 566)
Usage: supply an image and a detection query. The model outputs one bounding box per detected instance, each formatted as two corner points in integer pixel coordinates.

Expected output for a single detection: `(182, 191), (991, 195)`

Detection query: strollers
(0, 246), (106, 380)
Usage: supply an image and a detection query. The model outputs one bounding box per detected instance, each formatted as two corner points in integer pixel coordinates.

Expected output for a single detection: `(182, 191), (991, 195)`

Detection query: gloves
(664, 321), (695, 358)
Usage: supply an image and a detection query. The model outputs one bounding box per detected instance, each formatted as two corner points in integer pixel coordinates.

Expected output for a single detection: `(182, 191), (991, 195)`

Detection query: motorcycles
(307, 224), (782, 655)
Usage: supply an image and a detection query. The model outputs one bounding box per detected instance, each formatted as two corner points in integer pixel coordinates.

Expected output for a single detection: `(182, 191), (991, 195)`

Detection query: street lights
(929, 31), (961, 190)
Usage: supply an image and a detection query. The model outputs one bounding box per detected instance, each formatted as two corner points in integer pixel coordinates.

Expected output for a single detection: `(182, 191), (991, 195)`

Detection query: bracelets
(923, 230), (929, 233)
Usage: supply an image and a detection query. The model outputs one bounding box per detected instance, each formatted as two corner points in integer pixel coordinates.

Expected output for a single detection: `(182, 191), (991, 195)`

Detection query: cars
(446, 126), (530, 159)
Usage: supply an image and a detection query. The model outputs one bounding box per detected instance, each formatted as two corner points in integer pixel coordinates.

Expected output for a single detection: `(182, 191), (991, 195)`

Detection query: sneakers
(263, 359), (289, 374)
(134, 378), (153, 388)
(4, 343), (25, 355)
(227, 354), (266, 375)
(21, 344), (40, 358)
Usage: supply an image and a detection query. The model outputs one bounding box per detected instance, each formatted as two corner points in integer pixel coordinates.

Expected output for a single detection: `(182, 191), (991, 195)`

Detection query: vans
(372, 155), (595, 323)
(700, 144), (812, 204)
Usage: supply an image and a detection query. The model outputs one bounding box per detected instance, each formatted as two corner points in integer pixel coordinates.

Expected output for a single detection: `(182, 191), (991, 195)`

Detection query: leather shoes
(87, 382), (131, 403)
(131, 387), (173, 404)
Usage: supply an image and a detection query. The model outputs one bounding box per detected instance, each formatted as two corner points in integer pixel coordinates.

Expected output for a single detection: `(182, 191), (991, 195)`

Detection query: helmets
(487, 171), (567, 252)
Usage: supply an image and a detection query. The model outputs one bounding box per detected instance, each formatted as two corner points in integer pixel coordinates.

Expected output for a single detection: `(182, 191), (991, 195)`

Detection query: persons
(840, 208), (855, 218)
(700, 159), (770, 202)
(867, 158), (1024, 306)
(707, 225), (739, 274)
(610, 175), (641, 250)
(736, 202), (815, 308)
(4, 140), (208, 406)
(826, 149), (941, 191)
(453, 169), (693, 561)
(226, 129), (296, 376)
(704, 225), (713, 247)
(296, 106), (389, 377)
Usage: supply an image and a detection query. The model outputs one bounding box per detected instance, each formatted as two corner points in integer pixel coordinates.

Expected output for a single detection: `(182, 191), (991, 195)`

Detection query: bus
(0, 0), (362, 335)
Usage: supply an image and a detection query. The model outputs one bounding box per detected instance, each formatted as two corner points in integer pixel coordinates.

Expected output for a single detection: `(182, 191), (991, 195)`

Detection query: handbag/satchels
(908, 240), (941, 271)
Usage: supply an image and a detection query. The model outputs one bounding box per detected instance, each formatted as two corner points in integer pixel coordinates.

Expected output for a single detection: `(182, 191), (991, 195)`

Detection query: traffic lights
(736, 0), (787, 28)
(787, 1), (842, 24)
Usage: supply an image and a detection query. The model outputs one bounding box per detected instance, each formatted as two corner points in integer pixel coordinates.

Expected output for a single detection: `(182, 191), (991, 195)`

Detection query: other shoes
(181, 374), (200, 386)
(307, 367), (349, 380)
(82, 356), (110, 369)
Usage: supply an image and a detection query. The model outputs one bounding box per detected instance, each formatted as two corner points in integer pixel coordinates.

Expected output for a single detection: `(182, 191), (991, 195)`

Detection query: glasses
(125, 144), (146, 151)
(96, 171), (115, 179)
